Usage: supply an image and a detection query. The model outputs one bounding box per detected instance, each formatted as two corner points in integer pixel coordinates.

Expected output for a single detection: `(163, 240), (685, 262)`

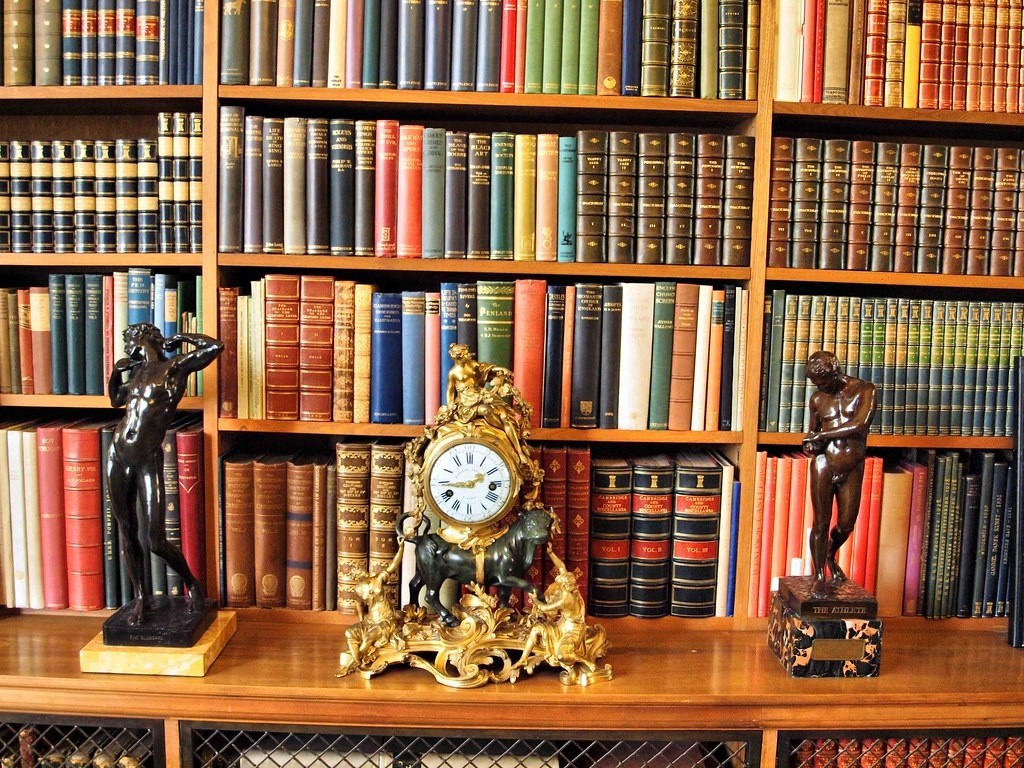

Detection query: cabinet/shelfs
(0, 0), (1024, 768)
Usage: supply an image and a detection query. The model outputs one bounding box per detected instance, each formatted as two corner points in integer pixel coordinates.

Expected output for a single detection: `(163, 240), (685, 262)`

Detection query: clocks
(333, 342), (617, 686)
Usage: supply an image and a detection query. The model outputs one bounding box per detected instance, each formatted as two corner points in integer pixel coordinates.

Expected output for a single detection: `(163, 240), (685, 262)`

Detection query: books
(219, 0), (759, 100)
(0, 267), (203, 397)
(217, 275), (750, 432)
(0, 414), (204, 611)
(0, 110), (203, 253)
(0, 723), (1024, 768)
(768, 135), (1024, 276)
(775, 0), (1024, 113)
(0, 0), (204, 87)
(748, 449), (1016, 617)
(226, 440), (742, 618)
(758, 289), (1024, 436)
(218, 104), (755, 268)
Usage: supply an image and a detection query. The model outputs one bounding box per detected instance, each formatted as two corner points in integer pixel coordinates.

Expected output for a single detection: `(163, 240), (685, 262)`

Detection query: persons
(802, 351), (877, 594)
(108, 323), (225, 626)
(335, 534), (409, 678)
(508, 541), (597, 673)
(446, 342), (527, 464)
